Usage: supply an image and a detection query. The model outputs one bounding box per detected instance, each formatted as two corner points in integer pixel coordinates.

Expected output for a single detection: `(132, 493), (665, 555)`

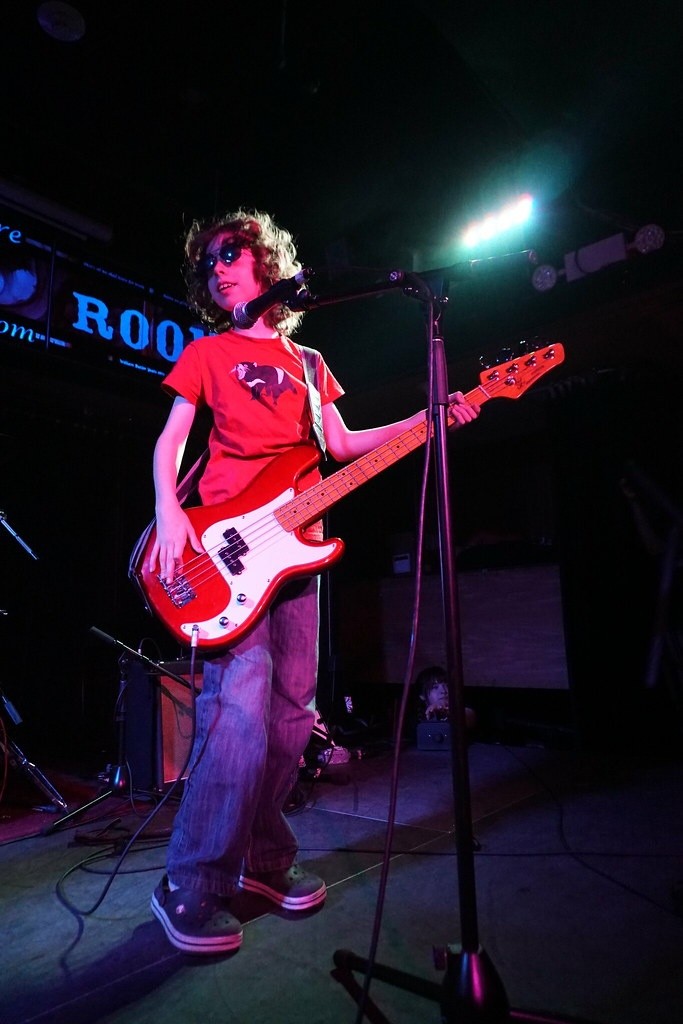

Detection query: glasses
(197, 242), (241, 273)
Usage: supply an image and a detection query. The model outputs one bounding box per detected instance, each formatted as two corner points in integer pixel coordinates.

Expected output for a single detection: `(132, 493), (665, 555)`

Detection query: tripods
(41, 626), (202, 837)
(293, 266), (589, 1024)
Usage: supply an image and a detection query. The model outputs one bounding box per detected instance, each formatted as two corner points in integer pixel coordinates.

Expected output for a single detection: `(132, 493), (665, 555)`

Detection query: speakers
(120, 656), (205, 797)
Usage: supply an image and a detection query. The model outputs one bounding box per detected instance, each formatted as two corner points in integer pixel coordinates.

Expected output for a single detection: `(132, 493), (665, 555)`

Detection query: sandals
(238, 858), (327, 910)
(151, 873), (243, 952)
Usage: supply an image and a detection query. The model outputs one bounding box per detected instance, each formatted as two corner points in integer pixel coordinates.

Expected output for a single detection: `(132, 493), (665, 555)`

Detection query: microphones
(231, 266), (315, 329)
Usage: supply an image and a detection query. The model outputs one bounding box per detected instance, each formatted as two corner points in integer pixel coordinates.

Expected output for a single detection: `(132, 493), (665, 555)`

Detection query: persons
(150, 210), (481, 952)
(415, 666), (475, 722)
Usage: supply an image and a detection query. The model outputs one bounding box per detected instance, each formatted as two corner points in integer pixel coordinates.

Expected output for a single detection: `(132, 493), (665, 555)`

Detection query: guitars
(131, 336), (570, 659)
